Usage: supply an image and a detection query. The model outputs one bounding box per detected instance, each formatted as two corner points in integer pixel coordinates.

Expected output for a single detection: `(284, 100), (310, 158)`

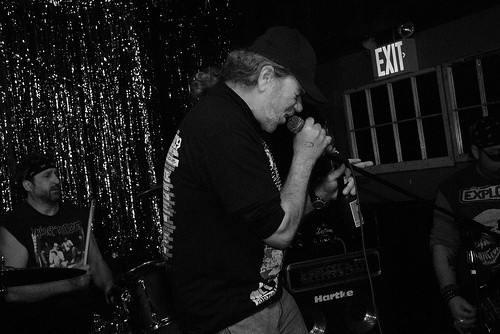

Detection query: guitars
(463, 269), (500, 334)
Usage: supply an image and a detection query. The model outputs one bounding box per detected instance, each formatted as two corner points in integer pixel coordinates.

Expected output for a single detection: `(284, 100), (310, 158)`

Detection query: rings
(460, 319), (464, 324)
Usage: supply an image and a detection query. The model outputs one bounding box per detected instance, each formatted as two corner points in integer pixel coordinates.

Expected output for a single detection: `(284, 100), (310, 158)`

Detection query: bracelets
(440, 283), (462, 304)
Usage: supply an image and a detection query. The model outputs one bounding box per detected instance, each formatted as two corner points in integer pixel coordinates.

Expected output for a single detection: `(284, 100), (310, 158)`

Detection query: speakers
(293, 275), (390, 334)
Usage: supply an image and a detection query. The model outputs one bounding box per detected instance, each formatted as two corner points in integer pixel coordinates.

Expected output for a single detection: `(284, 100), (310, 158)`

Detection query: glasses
(483, 150), (500, 162)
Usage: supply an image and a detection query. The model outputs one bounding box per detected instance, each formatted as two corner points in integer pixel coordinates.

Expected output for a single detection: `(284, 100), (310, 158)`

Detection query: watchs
(309, 188), (329, 209)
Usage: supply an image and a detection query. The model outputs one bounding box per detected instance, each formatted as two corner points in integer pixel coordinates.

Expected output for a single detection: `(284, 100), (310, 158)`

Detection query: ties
(250, 140), (286, 307)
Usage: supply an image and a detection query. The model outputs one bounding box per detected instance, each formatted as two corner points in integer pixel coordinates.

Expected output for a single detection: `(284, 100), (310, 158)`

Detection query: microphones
(287, 115), (340, 155)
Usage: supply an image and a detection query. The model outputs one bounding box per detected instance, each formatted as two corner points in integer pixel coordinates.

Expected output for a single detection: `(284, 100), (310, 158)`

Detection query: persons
(430, 116), (500, 334)
(0, 159), (120, 304)
(162, 26), (374, 334)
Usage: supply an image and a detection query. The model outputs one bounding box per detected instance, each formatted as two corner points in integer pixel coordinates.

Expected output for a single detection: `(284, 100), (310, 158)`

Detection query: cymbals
(137, 184), (163, 199)
(0, 267), (87, 289)
(106, 260), (163, 303)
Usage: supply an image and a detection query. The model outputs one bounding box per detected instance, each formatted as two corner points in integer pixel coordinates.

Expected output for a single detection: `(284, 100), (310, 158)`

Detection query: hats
(470, 117), (500, 150)
(229, 24), (329, 104)
(14, 151), (57, 181)
